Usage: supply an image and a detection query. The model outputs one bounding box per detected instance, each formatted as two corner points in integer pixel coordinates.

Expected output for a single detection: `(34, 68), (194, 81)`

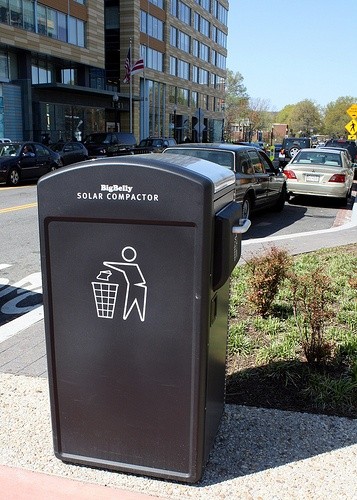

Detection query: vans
(82, 131), (137, 157)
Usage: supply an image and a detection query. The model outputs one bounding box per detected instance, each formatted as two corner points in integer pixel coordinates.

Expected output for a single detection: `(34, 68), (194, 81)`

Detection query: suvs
(127, 136), (177, 155)
(278, 137), (311, 166)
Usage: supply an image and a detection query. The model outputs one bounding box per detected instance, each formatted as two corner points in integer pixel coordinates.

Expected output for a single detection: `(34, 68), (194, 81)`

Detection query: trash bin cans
(36, 153), (251, 484)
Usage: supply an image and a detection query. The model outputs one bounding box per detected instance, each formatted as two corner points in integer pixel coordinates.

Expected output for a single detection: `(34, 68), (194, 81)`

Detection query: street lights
(172, 104), (178, 139)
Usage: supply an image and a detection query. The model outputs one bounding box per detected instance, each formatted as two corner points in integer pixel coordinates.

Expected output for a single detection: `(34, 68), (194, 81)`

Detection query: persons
(248, 139), (264, 146)
(278, 148), (288, 167)
(73, 127), (88, 147)
(23, 146), (36, 156)
(42, 136), (64, 146)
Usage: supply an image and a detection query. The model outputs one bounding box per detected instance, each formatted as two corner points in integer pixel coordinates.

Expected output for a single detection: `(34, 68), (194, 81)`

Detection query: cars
(161, 142), (289, 224)
(271, 143), (282, 152)
(51, 142), (88, 166)
(324, 138), (357, 164)
(0, 140), (64, 187)
(280, 146), (355, 200)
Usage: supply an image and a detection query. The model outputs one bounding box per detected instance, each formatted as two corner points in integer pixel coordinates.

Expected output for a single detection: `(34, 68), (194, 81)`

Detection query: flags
(122, 46), (144, 84)
